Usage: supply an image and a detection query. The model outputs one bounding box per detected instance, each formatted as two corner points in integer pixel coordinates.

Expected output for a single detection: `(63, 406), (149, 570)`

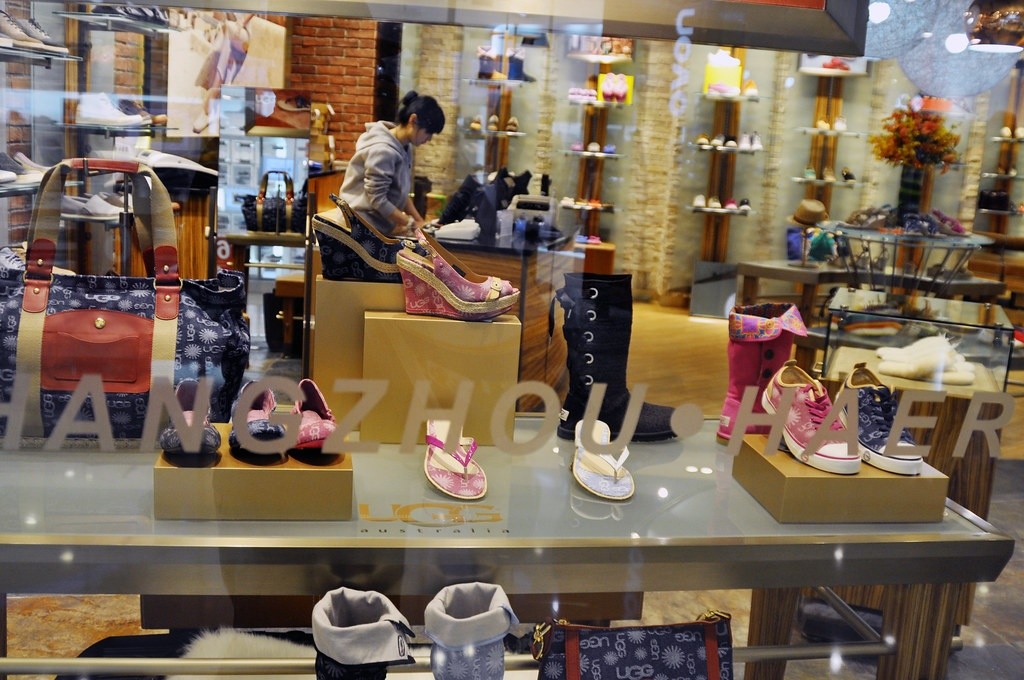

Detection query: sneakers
(0, 3), (180, 281)
(835, 362), (924, 476)
(435, 27), (1023, 296)
(761, 359), (861, 474)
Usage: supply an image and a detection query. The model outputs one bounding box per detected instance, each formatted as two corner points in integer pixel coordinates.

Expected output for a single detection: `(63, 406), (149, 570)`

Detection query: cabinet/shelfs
(0, 45), (1024, 680)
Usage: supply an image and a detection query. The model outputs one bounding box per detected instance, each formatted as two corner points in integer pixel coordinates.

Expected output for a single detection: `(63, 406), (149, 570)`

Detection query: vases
(895, 164), (925, 226)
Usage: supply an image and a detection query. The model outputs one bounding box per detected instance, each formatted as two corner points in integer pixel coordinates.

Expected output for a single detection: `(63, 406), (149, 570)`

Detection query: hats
(788, 198), (830, 228)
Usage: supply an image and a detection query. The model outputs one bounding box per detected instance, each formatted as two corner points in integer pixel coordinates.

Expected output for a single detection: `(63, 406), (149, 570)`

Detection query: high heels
(395, 227), (520, 320)
(311, 194), (431, 284)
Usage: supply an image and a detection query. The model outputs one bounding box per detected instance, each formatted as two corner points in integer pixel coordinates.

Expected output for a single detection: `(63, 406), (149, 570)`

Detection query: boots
(425, 582), (520, 680)
(714, 302), (807, 446)
(310, 587), (416, 680)
(548, 273), (678, 443)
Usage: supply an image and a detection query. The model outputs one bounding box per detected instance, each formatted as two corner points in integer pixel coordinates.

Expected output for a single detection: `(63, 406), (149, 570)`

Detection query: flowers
(868, 97), (962, 174)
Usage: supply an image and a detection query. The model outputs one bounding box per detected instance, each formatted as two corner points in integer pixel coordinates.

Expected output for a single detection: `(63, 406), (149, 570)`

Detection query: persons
(339, 91), (444, 235)
(194, 11), (249, 133)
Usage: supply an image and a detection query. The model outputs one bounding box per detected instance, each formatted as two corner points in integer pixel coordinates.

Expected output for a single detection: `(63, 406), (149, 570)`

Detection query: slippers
(572, 418), (635, 501)
(277, 96), (310, 111)
(423, 420), (487, 501)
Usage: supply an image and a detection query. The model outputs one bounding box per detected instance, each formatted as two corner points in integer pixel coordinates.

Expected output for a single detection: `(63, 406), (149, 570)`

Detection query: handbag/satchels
(0, 156), (250, 438)
(527, 606), (732, 680)
(241, 170), (308, 235)
(263, 287), (305, 350)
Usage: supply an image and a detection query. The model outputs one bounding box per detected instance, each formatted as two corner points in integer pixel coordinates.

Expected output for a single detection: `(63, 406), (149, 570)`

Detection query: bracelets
(407, 216), (414, 227)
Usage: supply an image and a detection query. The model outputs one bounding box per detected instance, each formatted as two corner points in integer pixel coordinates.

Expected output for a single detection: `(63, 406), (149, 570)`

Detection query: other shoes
(159, 378), (221, 455)
(229, 380), (284, 462)
(283, 379), (341, 454)
